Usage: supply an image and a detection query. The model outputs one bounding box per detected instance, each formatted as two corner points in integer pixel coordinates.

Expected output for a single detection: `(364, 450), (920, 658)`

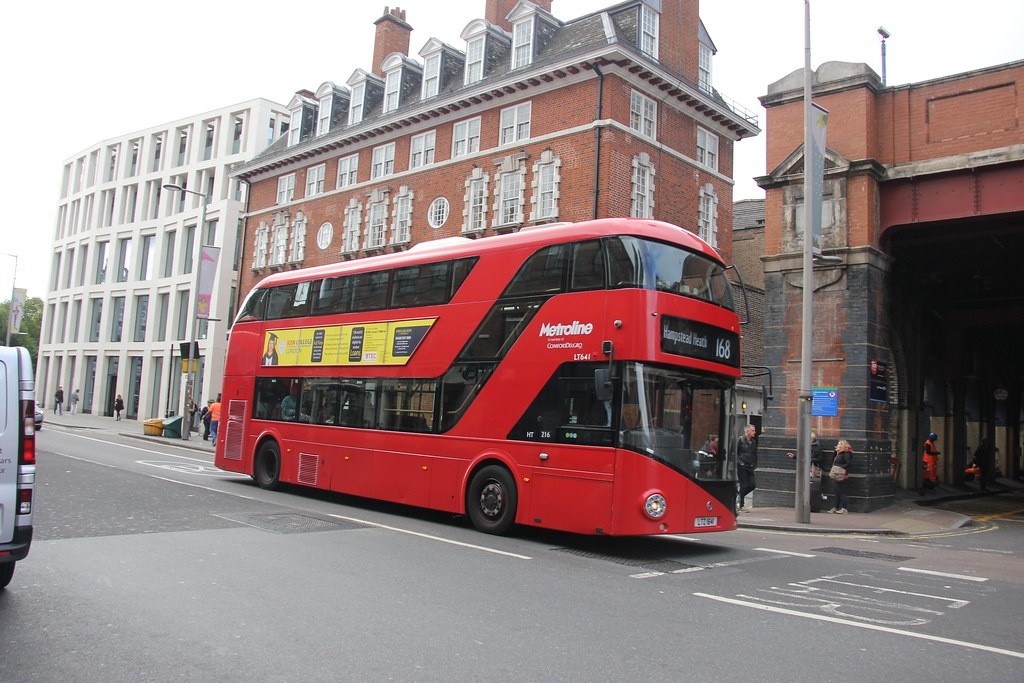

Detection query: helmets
(928, 433), (938, 440)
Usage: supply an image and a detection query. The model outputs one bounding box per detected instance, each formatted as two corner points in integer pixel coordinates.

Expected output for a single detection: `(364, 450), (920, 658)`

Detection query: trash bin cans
(162, 416), (183, 438)
(143, 418), (163, 436)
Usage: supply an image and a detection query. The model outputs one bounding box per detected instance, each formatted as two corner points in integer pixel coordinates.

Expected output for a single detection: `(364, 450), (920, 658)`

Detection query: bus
(214, 217), (774, 537)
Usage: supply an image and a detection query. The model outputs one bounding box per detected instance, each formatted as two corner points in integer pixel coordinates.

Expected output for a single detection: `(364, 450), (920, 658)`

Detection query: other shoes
(739, 506), (750, 512)
(811, 506), (820, 512)
(826, 507), (838, 513)
(213, 441), (216, 446)
(836, 507), (848, 514)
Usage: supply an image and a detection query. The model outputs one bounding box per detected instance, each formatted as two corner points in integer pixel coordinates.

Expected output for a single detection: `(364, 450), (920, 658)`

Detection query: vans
(0, 345), (38, 588)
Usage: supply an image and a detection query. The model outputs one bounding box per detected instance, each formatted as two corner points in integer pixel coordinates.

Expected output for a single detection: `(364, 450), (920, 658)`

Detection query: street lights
(164, 184), (207, 440)
(794, 253), (843, 524)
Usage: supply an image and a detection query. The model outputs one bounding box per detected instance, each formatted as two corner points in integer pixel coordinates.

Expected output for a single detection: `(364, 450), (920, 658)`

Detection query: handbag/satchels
(121, 405), (124, 409)
(829, 465), (847, 481)
(810, 462), (822, 492)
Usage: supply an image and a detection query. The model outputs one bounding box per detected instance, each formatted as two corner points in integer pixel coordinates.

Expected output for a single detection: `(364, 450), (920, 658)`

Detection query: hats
(269, 332), (279, 344)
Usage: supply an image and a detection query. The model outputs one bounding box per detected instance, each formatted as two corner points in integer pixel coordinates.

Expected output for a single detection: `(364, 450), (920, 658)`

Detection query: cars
(34, 402), (44, 431)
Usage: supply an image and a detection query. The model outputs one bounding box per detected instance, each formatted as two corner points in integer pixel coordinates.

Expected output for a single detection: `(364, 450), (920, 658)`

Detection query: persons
(735, 424), (757, 512)
(786, 433), (823, 513)
(323, 409), (336, 424)
(827, 440), (853, 514)
(696, 435), (723, 479)
(70, 389), (80, 415)
(112, 394), (124, 421)
(189, 398), (221, 446)
(280, 384), (309, 423)
(922, 433), (990, 488)
(53, 386), (64, 415)
(260, 332), (279, 366)
